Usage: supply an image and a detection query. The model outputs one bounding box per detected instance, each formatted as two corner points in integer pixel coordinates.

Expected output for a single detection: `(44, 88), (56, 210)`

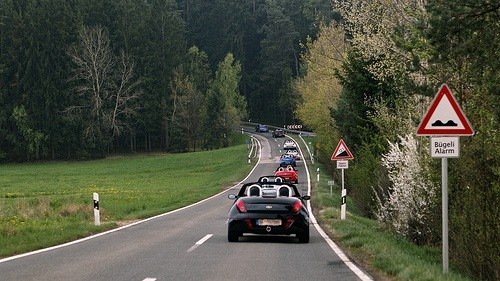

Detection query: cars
(272, 128), (286, 138)
(227, 149), (311, 244)
(254, 124), (270, 133)
(283, 139), (297, 150)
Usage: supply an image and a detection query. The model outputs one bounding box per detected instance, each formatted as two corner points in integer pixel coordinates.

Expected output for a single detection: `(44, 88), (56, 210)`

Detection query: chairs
(279, 167), (284, 171)
(277, 184), (292, 198)
(261, 177), (269, 182)
(247, 184), (263, 198)
(287, 165), (293, 171)
(275, 177), (282, 183)
